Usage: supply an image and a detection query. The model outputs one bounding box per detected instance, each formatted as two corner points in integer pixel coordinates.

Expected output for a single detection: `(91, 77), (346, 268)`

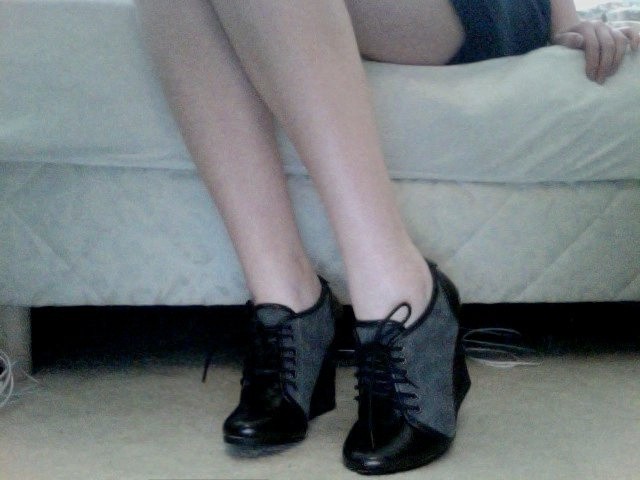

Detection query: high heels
(342, 257), (472, 476)
(201, 274), (361, 447)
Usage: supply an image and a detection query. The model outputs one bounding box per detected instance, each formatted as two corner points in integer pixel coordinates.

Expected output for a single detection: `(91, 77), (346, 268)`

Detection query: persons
(131, 0), (640, 475)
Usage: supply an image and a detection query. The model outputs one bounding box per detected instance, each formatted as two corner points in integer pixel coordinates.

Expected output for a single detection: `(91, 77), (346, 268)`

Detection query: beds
(1, 2), (639, 379)
